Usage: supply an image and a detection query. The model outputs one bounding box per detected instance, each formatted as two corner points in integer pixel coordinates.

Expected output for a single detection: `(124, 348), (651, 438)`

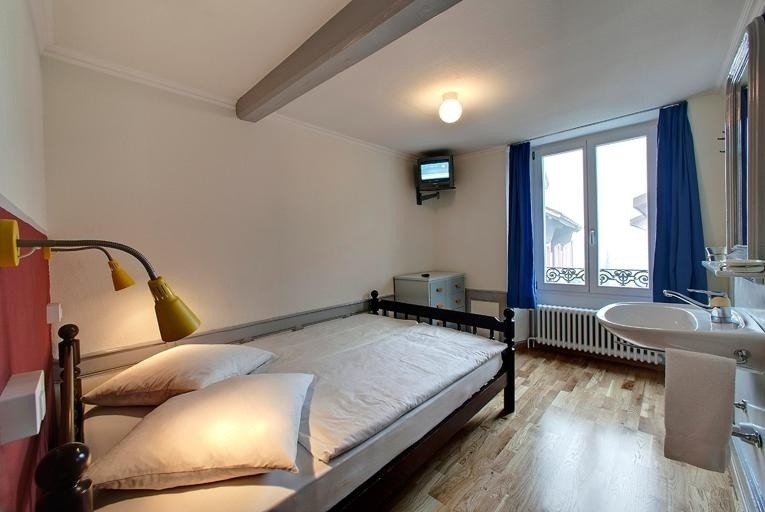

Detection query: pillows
(82, 372), (316, 492)
(78, 343), (281, 409)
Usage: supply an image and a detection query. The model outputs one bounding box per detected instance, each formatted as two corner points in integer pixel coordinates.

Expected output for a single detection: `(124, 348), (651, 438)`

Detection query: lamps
(18, 245), (137, 292)
(435, 89), (464, 127)
(1, 219), (201, 344)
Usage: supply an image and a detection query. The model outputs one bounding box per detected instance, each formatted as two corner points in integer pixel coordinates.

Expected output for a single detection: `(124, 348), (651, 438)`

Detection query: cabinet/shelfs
(392, 270), (469, 335)
(701, 258), (765, 287)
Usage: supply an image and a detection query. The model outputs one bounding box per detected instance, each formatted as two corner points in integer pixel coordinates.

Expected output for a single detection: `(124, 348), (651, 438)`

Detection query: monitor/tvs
(417, 155), (454, 191)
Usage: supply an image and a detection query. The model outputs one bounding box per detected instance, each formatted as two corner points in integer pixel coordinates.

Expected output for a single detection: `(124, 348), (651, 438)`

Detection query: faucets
(662, 288), (731, 324)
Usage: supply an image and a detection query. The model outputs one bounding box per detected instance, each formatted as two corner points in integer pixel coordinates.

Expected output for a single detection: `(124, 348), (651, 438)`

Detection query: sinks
(596, 301), (765, 376)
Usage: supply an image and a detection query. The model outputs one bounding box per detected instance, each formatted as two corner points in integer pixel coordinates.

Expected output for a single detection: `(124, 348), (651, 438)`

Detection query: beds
(33, 288), (516, 512)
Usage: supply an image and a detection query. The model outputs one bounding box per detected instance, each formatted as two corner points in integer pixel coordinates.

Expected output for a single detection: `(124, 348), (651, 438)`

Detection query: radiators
(526, 303), (666, 369)
